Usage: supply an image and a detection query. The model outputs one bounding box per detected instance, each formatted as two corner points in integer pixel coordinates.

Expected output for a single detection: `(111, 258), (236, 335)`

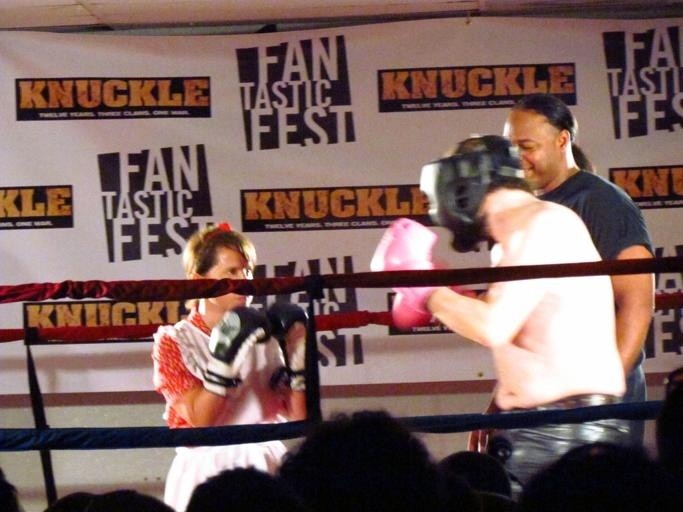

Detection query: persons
(41, 488), (177, 512)
(151, 220), (315, 512)
(464, 91), (656, 454)
(371, 134), (632, 496)
(188, 406), (511, 512)
(521, 443), (681, 512)
(1, 465), (27, 511)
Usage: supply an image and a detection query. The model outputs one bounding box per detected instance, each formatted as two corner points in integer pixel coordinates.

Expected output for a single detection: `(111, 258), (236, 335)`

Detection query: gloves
(266, 303), (308, 392)
(368, 216), (478, 328)
(202, 305), (270, 397)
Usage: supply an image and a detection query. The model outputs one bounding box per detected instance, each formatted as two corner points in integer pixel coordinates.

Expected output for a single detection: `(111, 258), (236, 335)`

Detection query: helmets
(419, 149), (524, 253)
